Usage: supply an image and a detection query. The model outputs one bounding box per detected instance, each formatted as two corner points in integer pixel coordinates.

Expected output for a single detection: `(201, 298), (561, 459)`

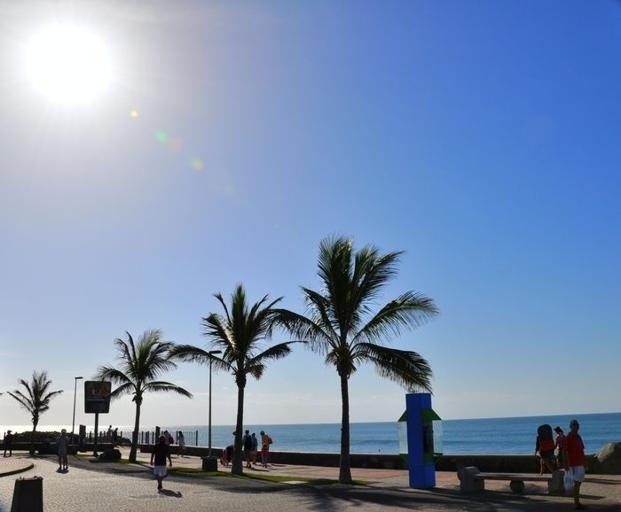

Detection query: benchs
(458, 466), (565, 496)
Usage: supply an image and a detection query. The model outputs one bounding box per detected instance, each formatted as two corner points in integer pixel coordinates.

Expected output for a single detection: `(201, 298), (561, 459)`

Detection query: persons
(220, 430), (272, 468)
(113, 428), (118, 447)
(164, 431), (172, 466)
(176, 431), (184, 457)
(57, 429), (68, 470)
(3, 430), (12, 457)
(150, 435), (172, 489)
(107, 425), (113, 440)
(534, 420), (586, 510)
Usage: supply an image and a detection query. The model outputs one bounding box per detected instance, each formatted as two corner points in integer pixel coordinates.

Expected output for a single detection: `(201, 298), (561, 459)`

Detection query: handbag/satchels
(563, 467), (576, 490)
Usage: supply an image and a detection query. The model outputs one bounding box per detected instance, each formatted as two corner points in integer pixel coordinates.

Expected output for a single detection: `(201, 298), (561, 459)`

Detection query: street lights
(72, 376), (83, 433)
(208, 350), (222, 459)
